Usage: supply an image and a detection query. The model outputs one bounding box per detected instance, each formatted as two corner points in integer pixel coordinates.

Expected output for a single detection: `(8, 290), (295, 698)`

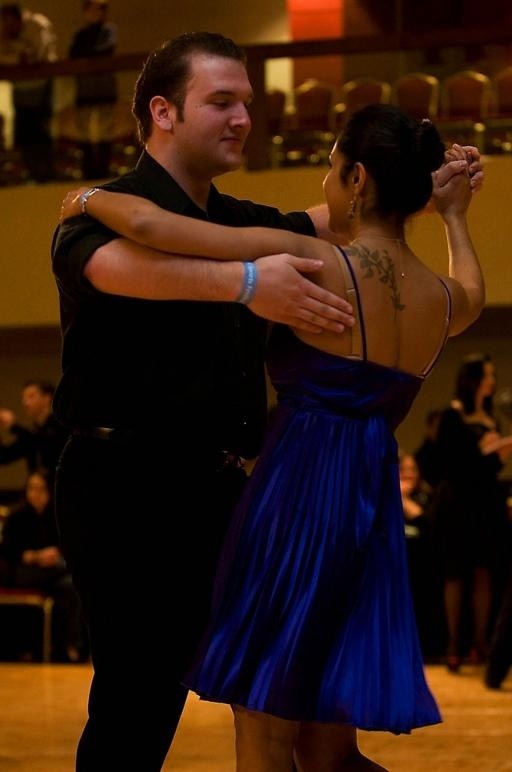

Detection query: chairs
(435, 66), (512, 153)
(242, 80), (337, 173)
(337, 73), (434, 124)
(0, 501), (67, 661)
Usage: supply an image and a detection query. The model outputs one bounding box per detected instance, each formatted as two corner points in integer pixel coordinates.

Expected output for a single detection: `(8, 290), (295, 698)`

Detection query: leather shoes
(440, 650), (509, 690)
